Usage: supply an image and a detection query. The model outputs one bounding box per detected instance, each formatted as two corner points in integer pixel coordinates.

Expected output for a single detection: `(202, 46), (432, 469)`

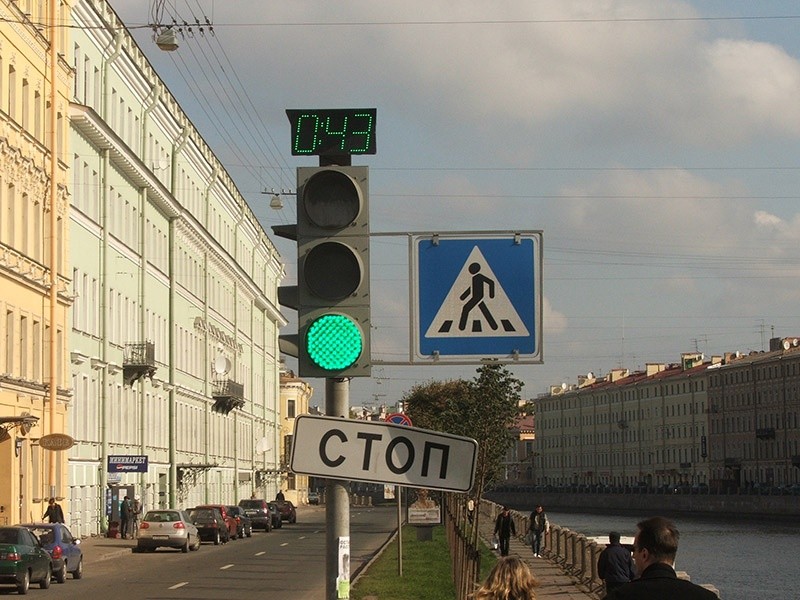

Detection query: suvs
(237, 499), (273, 532)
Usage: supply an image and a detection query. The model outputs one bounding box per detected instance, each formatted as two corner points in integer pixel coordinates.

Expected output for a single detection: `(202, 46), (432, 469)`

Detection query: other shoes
(534, 553), (537, 557)
(538, 554), (542, 558)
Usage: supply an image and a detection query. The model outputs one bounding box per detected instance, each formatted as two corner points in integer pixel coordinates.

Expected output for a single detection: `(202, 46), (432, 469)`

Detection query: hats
(502, 506), (510, 510)
(124, 496), (130, 499)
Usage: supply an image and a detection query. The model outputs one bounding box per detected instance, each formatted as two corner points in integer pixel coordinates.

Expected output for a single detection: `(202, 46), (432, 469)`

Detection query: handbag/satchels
(489, 534), (499, 550)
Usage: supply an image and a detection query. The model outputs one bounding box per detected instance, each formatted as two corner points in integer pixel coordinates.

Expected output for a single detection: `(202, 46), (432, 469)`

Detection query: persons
(41, 497), (65, 525)
(467, 500), (474, 526)
(597, 531), (637, 595)
(120, 494), (144, 540)
(492, 506), (516, 557)
(526, 505), (549, 558)
(601, 518), (720, 600)
(0, 505), (9, 526)
(467, 555), (540, 600)
(275, 490), (285, 501)
(410, 488), (436, 509)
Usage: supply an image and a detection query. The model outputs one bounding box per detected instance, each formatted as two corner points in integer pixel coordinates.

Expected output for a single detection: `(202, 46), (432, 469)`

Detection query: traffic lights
(295, 164), (374, 379)
(270, 224), (300, 359)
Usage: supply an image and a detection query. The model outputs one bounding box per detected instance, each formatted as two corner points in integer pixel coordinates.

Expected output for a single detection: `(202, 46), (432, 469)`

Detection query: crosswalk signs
(406, 233), (543, 364)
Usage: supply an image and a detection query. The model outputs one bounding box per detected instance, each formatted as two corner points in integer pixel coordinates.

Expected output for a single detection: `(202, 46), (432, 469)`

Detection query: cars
(270, 500), (298, 524)
(15, 524), (84, 584)
(196, 505), (239, 540)
(228, 505), (254, 539)
(0, 525), (54, 595)
(136, 508), (201, 553)
(184, 507), (231, 545)
(267, 503), (284, 529)
